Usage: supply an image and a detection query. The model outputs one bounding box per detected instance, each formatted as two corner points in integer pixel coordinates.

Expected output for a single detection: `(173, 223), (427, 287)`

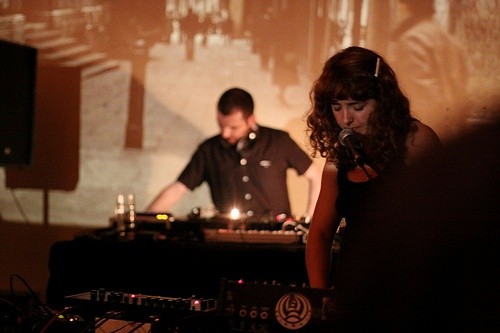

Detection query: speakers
(1, 39), (37, 164)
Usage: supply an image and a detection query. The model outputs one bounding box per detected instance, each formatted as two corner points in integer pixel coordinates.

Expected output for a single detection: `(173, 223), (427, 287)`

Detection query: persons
(304, 46), (441, 290)
(144, 88), (322, 222)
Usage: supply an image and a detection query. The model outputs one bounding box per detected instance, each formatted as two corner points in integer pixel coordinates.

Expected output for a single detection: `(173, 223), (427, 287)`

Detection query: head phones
(219, 123), (263, 157)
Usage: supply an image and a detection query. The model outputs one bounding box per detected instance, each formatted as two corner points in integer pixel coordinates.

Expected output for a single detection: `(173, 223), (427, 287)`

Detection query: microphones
(339, 128), (365, 170)
